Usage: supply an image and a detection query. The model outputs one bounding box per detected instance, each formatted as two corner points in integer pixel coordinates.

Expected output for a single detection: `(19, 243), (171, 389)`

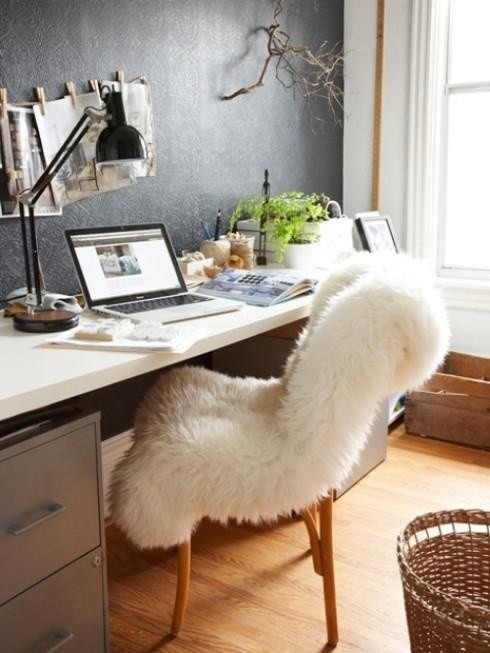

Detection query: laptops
(63, 221), (245, 325)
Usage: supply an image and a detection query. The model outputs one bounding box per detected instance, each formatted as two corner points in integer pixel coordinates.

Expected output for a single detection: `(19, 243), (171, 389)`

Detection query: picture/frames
(355, 215), (398, 253)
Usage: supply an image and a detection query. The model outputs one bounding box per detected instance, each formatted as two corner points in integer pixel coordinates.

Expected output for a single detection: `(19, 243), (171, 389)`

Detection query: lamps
(15, 83), (148, 331)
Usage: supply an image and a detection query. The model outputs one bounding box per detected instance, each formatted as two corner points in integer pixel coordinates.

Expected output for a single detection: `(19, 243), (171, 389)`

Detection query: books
(196, 266), (321, 308)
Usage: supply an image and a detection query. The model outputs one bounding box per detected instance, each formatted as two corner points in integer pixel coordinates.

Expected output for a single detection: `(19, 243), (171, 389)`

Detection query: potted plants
(223, 191), (325, 270)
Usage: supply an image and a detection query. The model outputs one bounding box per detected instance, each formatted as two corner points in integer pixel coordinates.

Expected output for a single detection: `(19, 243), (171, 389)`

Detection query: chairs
(108, 250), (451, 646)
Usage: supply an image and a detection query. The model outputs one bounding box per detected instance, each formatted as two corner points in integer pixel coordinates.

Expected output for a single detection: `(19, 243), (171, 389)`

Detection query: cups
(228, 236), (255, 270)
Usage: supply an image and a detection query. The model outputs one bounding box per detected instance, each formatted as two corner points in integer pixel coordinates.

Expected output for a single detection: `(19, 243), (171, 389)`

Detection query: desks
(0, 263), (312, 649)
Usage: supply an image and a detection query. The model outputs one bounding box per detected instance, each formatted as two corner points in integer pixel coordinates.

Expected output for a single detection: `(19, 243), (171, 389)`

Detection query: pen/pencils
(214, 209), (222, 241)
(201, 222), (212, 240)
